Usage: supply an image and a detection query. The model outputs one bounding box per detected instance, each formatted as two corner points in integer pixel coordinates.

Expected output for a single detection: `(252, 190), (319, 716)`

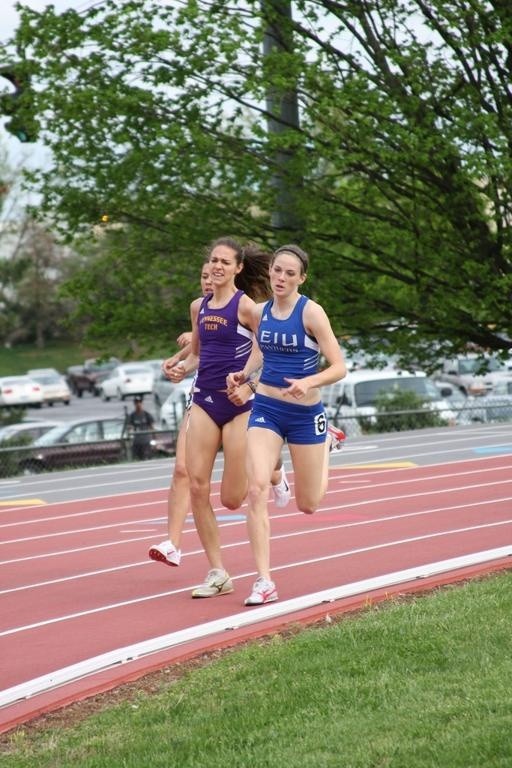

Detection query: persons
(226, 243), (347, 605)
(125, 394), (158, 441)
(147, 257), (214, 566)
(166, 236), (291, 598)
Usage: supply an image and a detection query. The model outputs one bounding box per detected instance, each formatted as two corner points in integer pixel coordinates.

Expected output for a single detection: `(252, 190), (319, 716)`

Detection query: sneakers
(191, 568), (235, 599)
(271, 454), (291, 509)
(245, 575), (280, 605)
(327, 423), (345, 451)
(147, 539), (182, 568)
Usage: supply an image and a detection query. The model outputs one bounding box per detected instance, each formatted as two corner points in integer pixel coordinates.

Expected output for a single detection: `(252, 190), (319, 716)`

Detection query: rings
(174, 373), (178, 377)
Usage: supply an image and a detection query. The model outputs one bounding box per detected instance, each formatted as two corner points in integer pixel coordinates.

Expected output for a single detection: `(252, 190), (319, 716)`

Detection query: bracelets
(247, 381), (257, 393)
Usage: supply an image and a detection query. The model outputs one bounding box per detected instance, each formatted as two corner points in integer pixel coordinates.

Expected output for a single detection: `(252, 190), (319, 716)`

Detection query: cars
(25, 365), (71, 409)
(0, 373), (43, 410)
(0, 418), (62, 447)
(14, 413), (178, 479)
